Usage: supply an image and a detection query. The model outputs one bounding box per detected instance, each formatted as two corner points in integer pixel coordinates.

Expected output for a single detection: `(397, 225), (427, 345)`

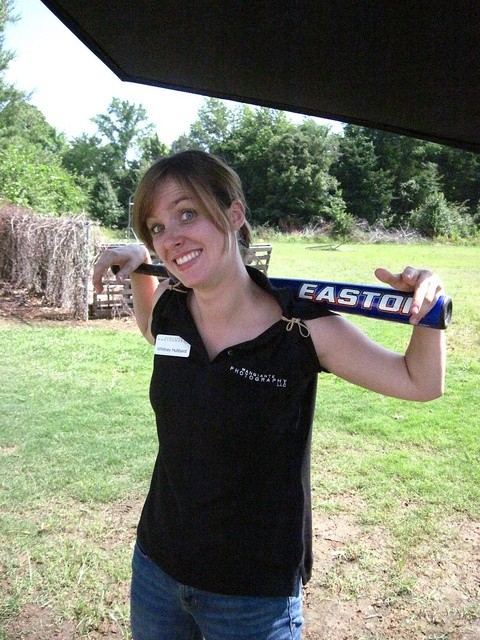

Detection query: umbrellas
(40, 1), (479, 158)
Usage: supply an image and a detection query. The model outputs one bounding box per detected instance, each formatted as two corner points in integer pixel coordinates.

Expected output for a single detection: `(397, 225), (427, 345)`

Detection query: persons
(89, 149), (448, 640)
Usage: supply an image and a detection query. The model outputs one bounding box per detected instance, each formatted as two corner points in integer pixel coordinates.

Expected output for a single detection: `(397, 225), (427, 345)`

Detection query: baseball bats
(111, 262), (452, 329)
